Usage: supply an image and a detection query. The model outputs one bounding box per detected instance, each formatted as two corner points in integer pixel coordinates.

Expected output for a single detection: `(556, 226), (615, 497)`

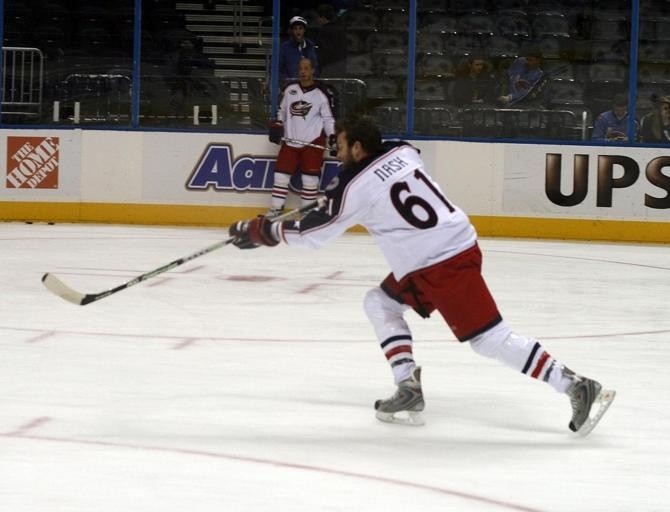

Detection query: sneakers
(561, 365), (600, 431)
(375, 367), (424, 410)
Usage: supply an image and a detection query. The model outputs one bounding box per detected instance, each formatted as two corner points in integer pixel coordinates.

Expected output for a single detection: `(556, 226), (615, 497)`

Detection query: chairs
(341, 4), (670, 143)
(2, 2), (232, 125)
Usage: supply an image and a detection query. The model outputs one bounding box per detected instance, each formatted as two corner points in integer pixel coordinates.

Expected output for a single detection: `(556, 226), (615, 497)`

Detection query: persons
(468, 47), (548, 109)
(589, 94), (668, 142)
(229, 116), (617, 434)
(278, 10), (345, 92)
(266, 54), (339, 219)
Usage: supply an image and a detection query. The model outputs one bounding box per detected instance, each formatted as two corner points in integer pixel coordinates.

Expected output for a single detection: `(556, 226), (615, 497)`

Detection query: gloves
(229, 215), (277, 249)
(329, 134), (338, 156)
(269, 121), (285, 143)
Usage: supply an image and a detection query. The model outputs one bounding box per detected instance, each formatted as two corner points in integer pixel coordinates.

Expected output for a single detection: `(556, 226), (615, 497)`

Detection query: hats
(290, 16), (307, 26)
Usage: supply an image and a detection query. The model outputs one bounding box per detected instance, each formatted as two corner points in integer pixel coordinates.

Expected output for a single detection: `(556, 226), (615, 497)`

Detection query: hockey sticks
(42, 198), (327, 305)
